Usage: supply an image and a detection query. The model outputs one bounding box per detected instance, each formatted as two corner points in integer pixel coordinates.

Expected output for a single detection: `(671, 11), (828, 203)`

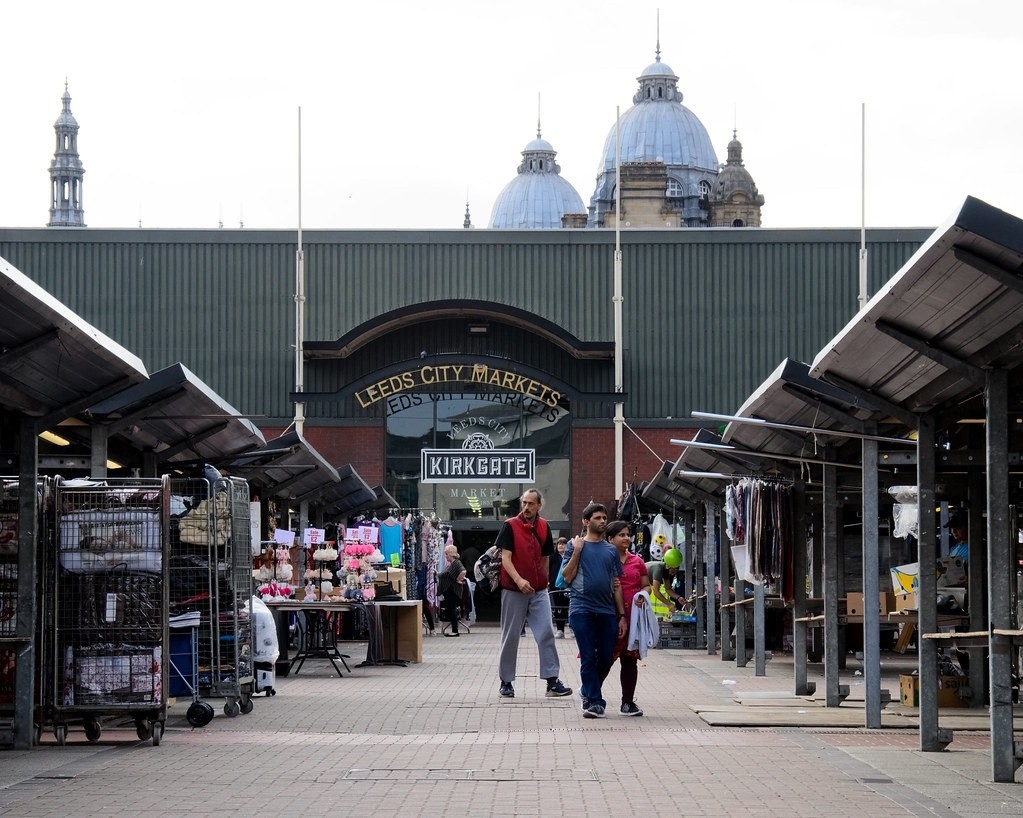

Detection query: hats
(943, 510), (968, 528)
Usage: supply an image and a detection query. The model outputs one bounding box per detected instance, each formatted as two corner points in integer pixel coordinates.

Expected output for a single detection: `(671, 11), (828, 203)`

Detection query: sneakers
(581, 700), (592, 711)
(620, 702), (643, 716)
(583, 705), (605, 717)
(579, 688), (589, 701)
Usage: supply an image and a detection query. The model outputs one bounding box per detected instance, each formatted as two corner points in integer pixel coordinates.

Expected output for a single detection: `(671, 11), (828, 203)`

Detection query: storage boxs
(899, 673), (968, 709)
(656, 621), (698, 649)
(847, 555), (969, 617)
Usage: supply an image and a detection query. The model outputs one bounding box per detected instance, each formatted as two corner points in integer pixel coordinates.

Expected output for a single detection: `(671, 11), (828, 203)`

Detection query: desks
(355, 600), (423, 664)
(277, 606), (352, 678)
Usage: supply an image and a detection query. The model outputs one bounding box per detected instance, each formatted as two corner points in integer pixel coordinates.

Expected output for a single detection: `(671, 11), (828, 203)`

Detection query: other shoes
(545, 679), (572, 697)
(444, 633), (459, 637)
(499, 682), (514, 697)
(429, 631), (436, 637)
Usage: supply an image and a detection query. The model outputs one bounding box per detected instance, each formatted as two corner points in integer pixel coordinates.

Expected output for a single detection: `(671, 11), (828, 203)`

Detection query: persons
(562, 501), (626, 716)
(494, 489), (573, 698)
(602, 520), (651, 716)
(437, 545), (466, 638)
(414, 569), (436, 636)
(642, 561), (687, 613)
(214, 595), (281, 665)
(943, 510), (988, 676)
(546, 536), (578, 640)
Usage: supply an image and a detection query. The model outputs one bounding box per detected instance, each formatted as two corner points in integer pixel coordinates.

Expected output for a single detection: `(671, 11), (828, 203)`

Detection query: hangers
(358, 508), (443, 534)
(726, 471), (794, 493)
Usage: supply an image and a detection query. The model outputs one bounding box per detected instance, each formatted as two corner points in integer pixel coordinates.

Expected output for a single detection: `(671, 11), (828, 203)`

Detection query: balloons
(664, 548), (684, 568)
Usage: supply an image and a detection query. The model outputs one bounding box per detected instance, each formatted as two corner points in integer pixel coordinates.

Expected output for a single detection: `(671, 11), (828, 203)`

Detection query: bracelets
(618, 614), (626, 618)
(676, 595), (682, 601)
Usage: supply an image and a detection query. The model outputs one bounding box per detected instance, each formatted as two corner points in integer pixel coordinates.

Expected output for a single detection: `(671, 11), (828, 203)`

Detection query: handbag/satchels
(474, 546), (502, 593)
(555, 558), (572, 589)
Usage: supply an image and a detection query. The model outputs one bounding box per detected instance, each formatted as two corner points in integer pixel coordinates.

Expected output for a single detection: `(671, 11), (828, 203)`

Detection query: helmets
(664, 549), (683, 568)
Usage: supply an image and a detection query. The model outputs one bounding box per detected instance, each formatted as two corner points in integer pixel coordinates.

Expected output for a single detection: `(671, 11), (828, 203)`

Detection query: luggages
(246, 611), (276, 697)
